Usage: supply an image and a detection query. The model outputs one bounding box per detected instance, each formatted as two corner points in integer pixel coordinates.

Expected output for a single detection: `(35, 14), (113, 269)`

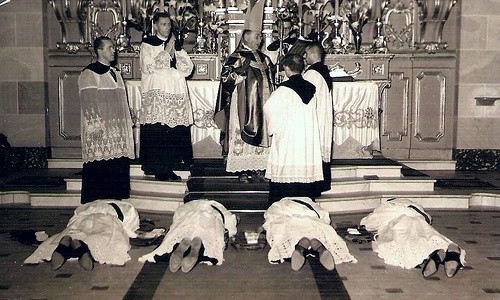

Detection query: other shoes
(144, 170), (156, 177)
(310, 238), (334, 271)
(446, 243), (461, 277)
(291, 237), (310, 272)
(155, 171), (182, 182)
(251, 171), (269, 183)
(169, 237), (191, 273)
(51, 235), (72, 270)
(422, 249), (445, 277)
(238, 173), (250, 184)
(72, 239), (94, 272)
(181, 237), (205, 273)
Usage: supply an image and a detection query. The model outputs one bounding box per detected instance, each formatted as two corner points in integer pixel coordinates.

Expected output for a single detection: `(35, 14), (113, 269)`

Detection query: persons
(360, 198), (467, 277)
(303, 43), (333, 197)
(24, 199), (139, 270)
(78, 36), (135, 204)
(139, 12), (194, 182)
(262, 54), (324, 204)
(139, 199), (240, 273)
(264, 196), (358, 271)
(214, 30), (274, 183)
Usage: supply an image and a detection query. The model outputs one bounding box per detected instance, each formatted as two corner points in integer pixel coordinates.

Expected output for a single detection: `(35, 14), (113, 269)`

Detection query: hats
(243, 0), (265, 32)
